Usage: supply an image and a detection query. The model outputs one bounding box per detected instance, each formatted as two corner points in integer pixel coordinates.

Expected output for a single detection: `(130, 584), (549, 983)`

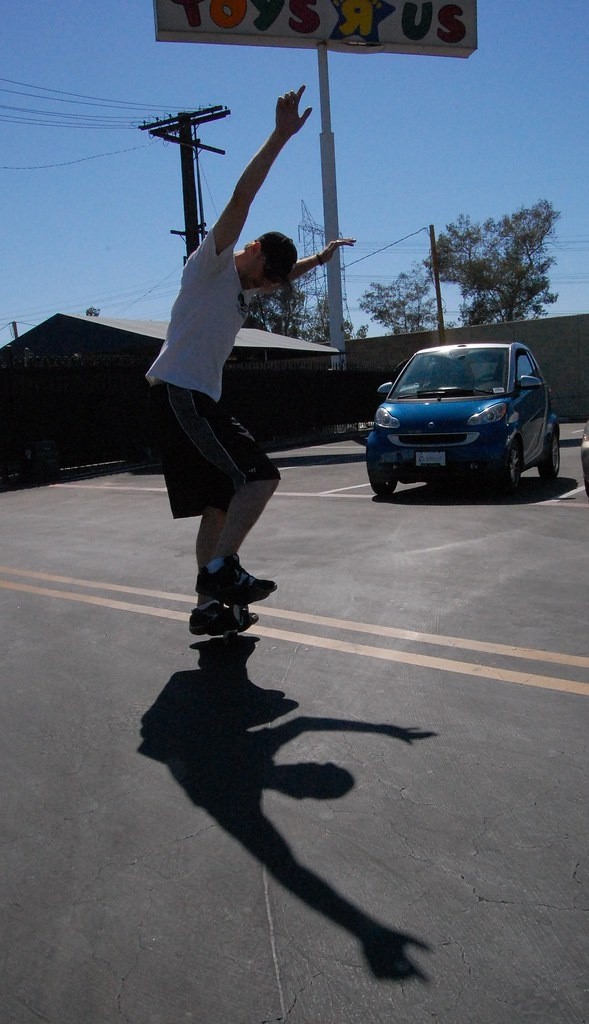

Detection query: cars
(366, 342), (561, 497)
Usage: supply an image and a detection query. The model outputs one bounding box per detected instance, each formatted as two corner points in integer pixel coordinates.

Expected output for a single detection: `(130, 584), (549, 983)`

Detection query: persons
(145, 86), (355, 646)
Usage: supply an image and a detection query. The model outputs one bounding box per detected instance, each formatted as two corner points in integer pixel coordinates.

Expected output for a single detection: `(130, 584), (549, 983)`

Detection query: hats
(259, 231), (297, 293)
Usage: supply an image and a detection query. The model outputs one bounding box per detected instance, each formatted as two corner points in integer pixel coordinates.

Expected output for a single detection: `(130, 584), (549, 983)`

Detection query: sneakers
(196, 553), (278, 606)
(189, 601), (259, 636)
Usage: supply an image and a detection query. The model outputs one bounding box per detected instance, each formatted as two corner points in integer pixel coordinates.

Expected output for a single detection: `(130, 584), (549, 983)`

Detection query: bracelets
(316, 253), (324, 266)
